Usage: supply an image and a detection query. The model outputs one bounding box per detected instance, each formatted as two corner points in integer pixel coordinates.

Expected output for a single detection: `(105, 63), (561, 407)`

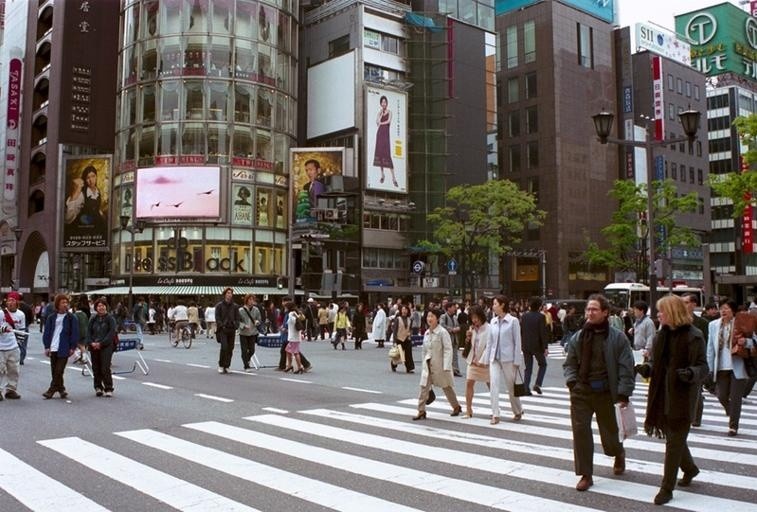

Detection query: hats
(7, 291), (19, 300)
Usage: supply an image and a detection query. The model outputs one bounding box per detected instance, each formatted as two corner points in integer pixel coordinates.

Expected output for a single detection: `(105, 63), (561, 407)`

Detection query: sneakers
(534, 385), (542, 394)
(218, 366), (232, 374)
(59, 390), (68, 398)
(95, 387), (103, 396)
(5, 390), (20, 399)
(275, 367), (283, 371)
(654, 488), (672, 504)
(306, 363), (312, 372)
(678, 465), (699, 486)
(43, 387), (54, 398)
(106, 392), (113, 397)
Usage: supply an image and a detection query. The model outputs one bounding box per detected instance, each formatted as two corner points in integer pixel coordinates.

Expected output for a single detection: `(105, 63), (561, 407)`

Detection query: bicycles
(168, 321), (192, 349)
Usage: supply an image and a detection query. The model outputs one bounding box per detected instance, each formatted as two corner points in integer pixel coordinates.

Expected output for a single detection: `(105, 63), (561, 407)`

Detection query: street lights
(120, 211), (148, 319)
(636, 217), (649, 285)
(591, 104), (702, 328)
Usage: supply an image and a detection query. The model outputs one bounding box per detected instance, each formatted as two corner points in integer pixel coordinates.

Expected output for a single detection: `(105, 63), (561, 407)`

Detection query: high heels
(451, 406), (462, 416)
(413, 412), (426, 420)
(284, 366), (293, 372)
(295, 366), (304, 373)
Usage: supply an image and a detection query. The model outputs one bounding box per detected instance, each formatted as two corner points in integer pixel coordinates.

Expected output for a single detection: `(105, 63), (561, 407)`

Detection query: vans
(539, 299), (586, 336)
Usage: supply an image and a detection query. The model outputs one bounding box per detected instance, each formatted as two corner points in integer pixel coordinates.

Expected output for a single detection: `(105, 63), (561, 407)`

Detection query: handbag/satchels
(462, 340), (471, 357)
(514, 382), (530, 396)
(295, 316), (304, 331)
(257, 321), (266, 334)
(389, 344), (406, 365)
(425, 390), (435, 404)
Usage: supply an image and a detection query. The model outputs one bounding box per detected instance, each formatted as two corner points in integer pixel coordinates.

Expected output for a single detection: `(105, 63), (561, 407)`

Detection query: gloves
(677, 368), (692, 383)
(635, 364), (650, 378)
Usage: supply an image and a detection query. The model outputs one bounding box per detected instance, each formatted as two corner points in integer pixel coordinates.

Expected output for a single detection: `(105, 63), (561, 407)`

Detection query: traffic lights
(276, 277), (288, 289)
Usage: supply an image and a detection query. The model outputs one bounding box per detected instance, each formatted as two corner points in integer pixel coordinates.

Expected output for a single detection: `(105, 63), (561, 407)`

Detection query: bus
(601, 279), (704, 321)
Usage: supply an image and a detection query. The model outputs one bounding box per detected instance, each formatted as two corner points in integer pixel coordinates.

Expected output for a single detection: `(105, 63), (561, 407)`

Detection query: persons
(371, 94), (399, 188)
(277, 200), (285, 215)
(371, 295), (566, 424)
(0, 287), (117, 400)
(563, 287), (756, 506)
(215, 288), (366, 374)
(299, 159), (324, 192)
(121, 188), (133, 210)
(101, 297), (217, 339)
(258, 197), (268, 213)
(234, 187), (251, 205)
(63, 165), (107, 237)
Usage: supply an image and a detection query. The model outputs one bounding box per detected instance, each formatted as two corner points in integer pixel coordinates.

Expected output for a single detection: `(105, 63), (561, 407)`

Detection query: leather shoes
(513, 413), (521, 420)
(490, 416), (499, 424)
(613, 456), (624, 474)
(728, 430), (737, 436)
(577, 476), (592, 490)
